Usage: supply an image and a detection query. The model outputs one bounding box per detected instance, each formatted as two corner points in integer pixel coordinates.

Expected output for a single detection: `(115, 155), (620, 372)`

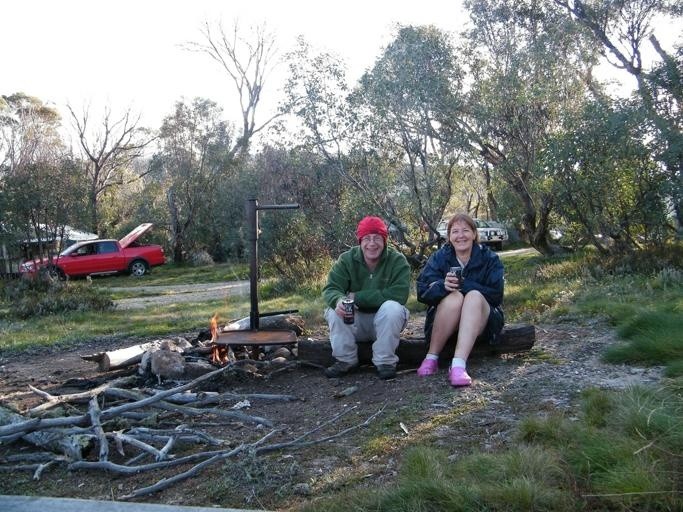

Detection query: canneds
(342, 299), (355, 324)
(450, 267), (463, 292)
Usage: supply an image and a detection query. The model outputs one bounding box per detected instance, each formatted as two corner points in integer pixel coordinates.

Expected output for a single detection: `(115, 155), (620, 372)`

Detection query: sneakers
(326, 362), (358, 377)
(417, 359), (438, 376)
(377, 364), (395, 378)
(449, 367), (471, 385)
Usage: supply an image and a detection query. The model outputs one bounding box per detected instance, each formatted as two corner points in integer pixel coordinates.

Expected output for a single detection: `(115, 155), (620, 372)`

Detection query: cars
(19, 222), (166, 285)
(432, 217), (509, 251)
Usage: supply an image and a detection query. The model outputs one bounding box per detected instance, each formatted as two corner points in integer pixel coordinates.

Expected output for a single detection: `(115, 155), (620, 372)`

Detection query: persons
(414, 212), (506, 386)
(320, 215), (410, 380)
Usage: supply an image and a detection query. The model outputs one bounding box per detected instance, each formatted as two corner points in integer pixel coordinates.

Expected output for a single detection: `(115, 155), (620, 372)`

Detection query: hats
(357, 217), (387, 243)
